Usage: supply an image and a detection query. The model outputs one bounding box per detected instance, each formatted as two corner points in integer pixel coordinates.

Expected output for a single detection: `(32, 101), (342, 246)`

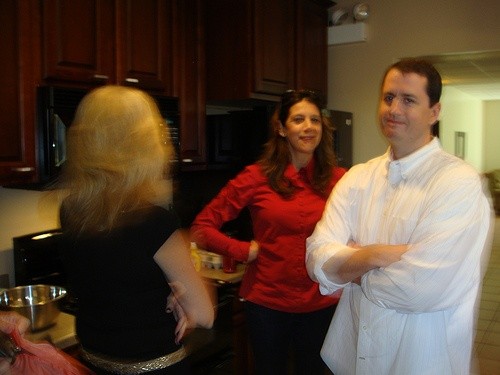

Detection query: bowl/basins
(0, 285), (67, 332)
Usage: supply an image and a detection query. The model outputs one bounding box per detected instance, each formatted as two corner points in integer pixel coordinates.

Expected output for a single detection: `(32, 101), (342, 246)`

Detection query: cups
(223, 255), (237, 273)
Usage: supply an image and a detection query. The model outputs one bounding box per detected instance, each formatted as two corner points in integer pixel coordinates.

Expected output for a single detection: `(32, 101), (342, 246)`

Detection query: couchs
(484, 169), (500, 218)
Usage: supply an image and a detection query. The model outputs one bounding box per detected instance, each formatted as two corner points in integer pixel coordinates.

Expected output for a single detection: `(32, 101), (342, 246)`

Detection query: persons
(305, 57), (491, 375)
(57, 84), (215, 375)
(189, 88), (349, 375)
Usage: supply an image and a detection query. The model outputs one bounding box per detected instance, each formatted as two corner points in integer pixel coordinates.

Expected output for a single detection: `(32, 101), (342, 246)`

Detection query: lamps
(328, 4), (370, 46)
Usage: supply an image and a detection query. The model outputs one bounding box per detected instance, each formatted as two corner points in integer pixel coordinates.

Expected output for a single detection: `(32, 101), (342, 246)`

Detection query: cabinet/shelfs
(192, 284), (254, 375)
(0, 1), (329, 191)
